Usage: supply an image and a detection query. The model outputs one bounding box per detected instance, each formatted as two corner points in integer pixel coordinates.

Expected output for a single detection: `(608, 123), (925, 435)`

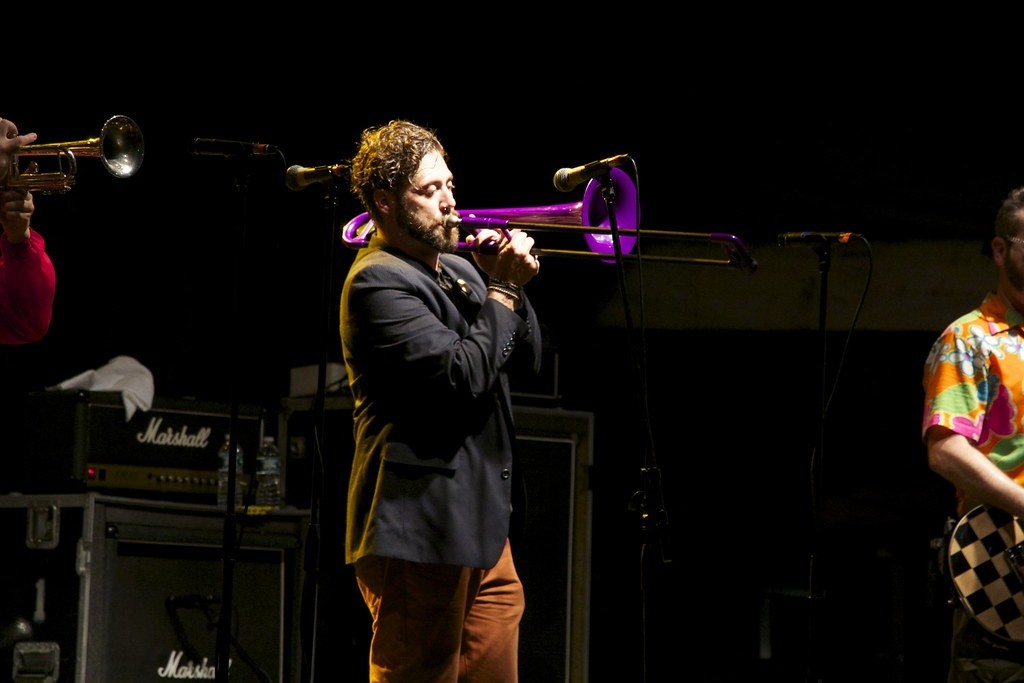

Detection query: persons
(923, 188), (1024, 683)
(0, 117), (56, 345)
(339, 119), (542, 683)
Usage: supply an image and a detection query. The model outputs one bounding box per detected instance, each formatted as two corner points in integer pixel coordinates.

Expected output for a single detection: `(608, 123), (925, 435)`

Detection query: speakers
(508, 434), (573, 682)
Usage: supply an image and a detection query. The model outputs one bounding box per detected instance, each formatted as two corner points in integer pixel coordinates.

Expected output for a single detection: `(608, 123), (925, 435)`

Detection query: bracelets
(487, 276), (520, 302)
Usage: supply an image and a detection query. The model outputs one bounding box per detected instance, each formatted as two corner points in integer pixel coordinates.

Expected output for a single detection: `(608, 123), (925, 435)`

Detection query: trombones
(339, 166), (748, 268)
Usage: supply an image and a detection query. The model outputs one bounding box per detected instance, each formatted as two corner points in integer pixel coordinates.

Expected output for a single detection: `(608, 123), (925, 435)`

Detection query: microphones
(551, 154), (627, 193)
(280, 160), (347, 187)
(776, 230), (841, 247)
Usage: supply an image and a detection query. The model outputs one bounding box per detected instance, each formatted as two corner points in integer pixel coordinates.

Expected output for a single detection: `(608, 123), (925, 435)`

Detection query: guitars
(946, 501), (1023, 644)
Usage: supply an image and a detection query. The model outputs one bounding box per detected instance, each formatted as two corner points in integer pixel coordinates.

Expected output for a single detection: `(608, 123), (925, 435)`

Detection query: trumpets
(0, 114), (146, 196)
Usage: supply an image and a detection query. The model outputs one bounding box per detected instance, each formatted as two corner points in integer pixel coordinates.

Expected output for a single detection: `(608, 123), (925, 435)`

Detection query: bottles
(255, 436), (283, 507)
(217, 434), (244, 510)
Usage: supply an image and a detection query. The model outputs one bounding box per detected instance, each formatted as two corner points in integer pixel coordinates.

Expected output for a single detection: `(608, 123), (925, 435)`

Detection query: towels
(47, 354), (156, 423)
